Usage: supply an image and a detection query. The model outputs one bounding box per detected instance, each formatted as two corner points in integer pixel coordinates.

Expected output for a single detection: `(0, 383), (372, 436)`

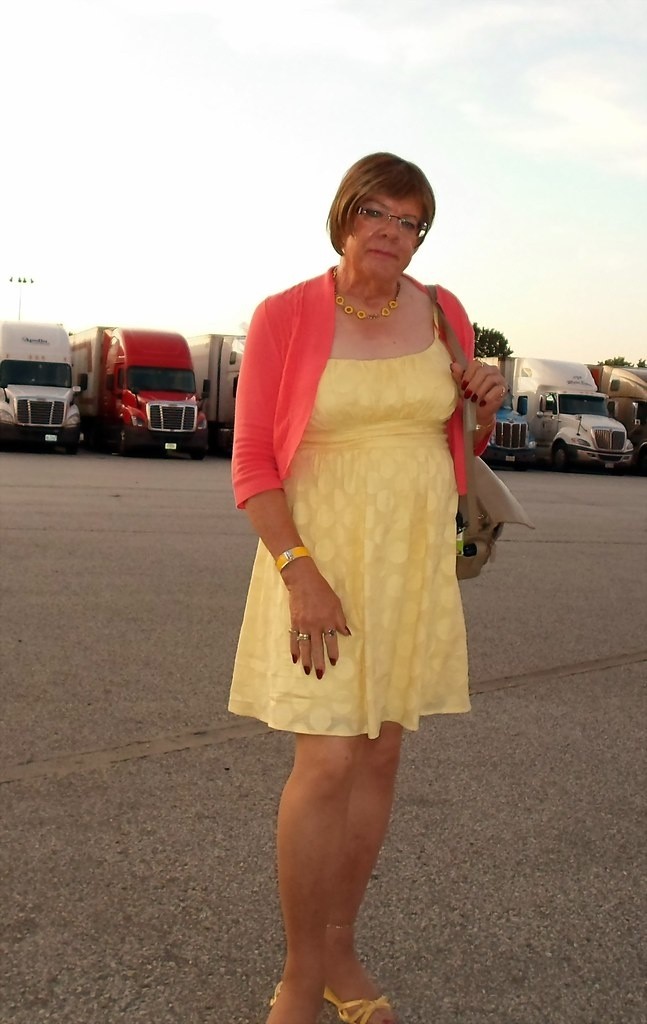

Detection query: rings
(287, 629), (298, 637)
(475, 357), (484, 367)
(297, 634), (310, 641)
(324, 629), (337, 637)
(501, 385), (507, 397)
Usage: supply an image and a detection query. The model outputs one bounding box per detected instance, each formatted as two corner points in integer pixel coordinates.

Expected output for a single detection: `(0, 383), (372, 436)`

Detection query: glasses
(356, 205), (429, 238)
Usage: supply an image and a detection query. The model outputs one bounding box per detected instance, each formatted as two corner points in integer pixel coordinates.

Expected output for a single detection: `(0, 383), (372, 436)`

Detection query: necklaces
(332, 267), (403, 321)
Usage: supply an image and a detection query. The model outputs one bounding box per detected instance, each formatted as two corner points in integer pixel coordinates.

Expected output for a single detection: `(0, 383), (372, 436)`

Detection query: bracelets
(273, 547), (315, 571)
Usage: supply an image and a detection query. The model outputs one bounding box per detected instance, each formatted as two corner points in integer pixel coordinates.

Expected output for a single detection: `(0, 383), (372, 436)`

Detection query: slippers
(323, 986), (393, 1024)
(270, 981), (284, 1007)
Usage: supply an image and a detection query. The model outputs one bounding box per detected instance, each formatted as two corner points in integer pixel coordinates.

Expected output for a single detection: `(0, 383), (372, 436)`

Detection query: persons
(233, 154), (533, 1024)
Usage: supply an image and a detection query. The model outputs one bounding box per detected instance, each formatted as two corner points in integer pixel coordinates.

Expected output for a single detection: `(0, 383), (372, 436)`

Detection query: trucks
(0, 320), (88, 455)
(184, 333), (250, 457)
(67, 326), (211, 461)
(478, 388), (539, 472)
(472, 356), (635, 476)
(585, 363), (647, 476)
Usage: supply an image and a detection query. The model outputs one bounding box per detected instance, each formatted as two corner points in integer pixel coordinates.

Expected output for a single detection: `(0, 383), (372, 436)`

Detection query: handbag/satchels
(456, 456), (535, 580)
